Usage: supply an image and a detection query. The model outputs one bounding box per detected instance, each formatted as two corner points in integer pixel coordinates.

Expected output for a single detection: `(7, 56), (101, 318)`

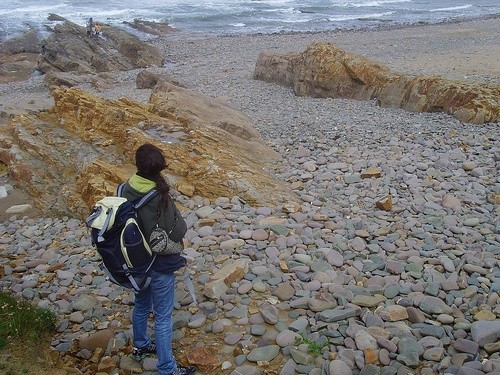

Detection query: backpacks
(84, 182), (161, 297)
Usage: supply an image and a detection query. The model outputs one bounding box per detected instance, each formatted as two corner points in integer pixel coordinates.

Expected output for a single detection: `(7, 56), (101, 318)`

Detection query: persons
(95, 23), (102, 34)
(116, 143), (198, 375)
(86, 18), (93, 38)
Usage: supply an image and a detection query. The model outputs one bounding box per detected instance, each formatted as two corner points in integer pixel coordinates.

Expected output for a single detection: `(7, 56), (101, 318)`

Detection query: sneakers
(132, 341), (157, 361)
(168, 365), (195, 375)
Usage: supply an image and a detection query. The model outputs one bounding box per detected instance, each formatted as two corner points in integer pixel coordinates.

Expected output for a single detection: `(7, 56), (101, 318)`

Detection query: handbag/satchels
(149, 191), (184, 254)
